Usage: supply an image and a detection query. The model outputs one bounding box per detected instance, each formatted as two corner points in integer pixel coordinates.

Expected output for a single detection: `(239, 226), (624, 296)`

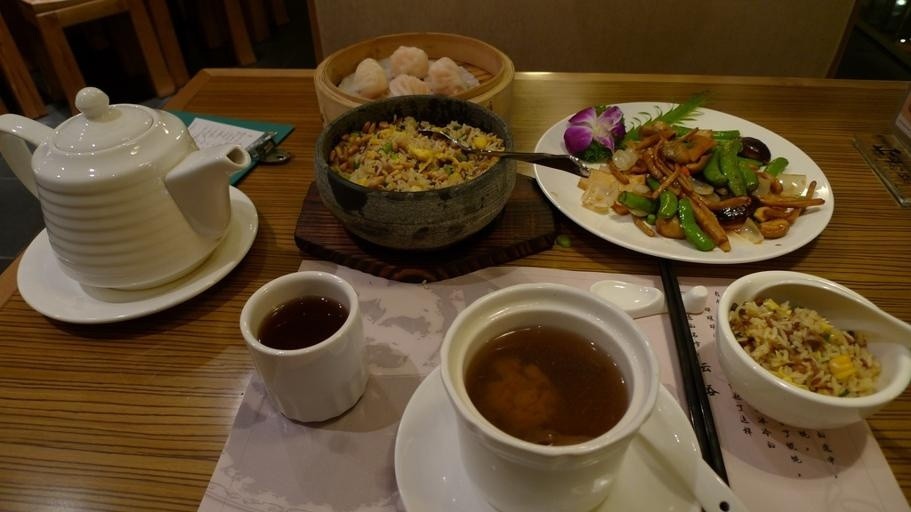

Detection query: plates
(394, 365), (704, 512)
(17, 185), (261, 325)
(532, 101), (835, 264)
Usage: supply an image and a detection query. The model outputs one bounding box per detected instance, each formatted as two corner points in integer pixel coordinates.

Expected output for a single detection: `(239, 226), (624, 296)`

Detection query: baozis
(349, 45), (469, 98)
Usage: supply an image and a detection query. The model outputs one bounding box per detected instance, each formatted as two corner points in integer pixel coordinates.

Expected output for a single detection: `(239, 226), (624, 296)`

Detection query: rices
(328, 116), (505, 193)
(729, 300), (882, 399)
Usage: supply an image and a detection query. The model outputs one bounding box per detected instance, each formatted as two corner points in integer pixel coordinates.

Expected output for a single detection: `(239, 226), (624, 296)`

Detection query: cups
(239, 272), (370, 425)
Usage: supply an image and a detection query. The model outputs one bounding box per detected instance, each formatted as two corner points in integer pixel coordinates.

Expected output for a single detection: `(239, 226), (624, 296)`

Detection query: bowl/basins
(439, 282), (661, 471)
(715, 270), (910, 431)
(315, 94), (517, 250)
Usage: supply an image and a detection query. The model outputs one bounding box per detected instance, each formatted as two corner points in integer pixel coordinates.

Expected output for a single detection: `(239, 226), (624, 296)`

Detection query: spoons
(752, 280), (910, 351)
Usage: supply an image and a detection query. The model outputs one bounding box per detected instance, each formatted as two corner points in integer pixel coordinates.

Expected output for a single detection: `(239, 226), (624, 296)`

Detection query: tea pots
(0, 87), (251, 291)
